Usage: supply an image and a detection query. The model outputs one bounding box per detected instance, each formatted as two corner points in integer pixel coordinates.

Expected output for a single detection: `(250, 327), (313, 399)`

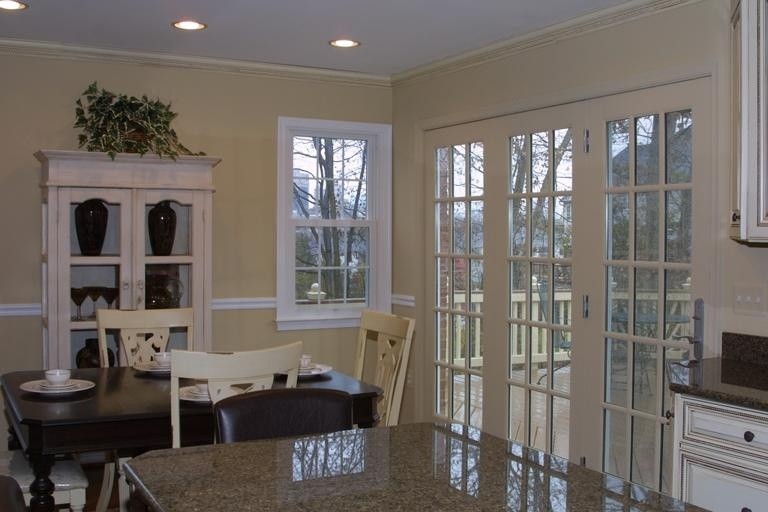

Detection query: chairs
(214, 387), (355, 443)
(352, 310), (416, 426)
(537, 278), (587, 387)
(170, 342), (302, 449)
(96, 308), (193, 369)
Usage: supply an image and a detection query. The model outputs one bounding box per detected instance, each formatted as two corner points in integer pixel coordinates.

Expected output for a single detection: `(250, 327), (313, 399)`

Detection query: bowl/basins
(45, 370), (70, 385)
(154, 352), (171, 365)
(301, 356), (312, 368)
(196, 384), (208, 392)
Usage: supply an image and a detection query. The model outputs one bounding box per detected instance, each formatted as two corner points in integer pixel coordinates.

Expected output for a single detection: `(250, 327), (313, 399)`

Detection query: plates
(280, 364), (332, 377)
(134, 361), (172, 375)
(190, 392), (209, 397)
(20, 380), (96, 395)
(179, 386), (211, 403)
(40, 384), (79, 390)
(150, 365), (171, 370)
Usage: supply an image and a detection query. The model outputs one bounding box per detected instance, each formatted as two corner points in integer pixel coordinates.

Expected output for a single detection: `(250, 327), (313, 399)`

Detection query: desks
(610, 311), (691, 393)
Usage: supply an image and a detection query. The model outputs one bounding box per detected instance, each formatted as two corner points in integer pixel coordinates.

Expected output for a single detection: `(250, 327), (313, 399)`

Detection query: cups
(71, 289), (89, 321)
(84, 287), (105, 318)
(103, 288), (119, 308)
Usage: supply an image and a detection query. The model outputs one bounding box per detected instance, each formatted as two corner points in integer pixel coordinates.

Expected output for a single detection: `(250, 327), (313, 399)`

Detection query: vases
(74, 339), (114, 370)
(75, 199), (108, 256)
(148, 201), (178, 255)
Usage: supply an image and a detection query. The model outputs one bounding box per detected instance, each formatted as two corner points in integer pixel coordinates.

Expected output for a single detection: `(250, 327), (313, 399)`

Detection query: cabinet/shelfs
(28, 151), (222, 371)
(668, 357), (764, 512)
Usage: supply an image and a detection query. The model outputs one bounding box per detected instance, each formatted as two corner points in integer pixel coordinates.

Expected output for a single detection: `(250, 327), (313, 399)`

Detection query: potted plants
(73, 83), (207, 161)
